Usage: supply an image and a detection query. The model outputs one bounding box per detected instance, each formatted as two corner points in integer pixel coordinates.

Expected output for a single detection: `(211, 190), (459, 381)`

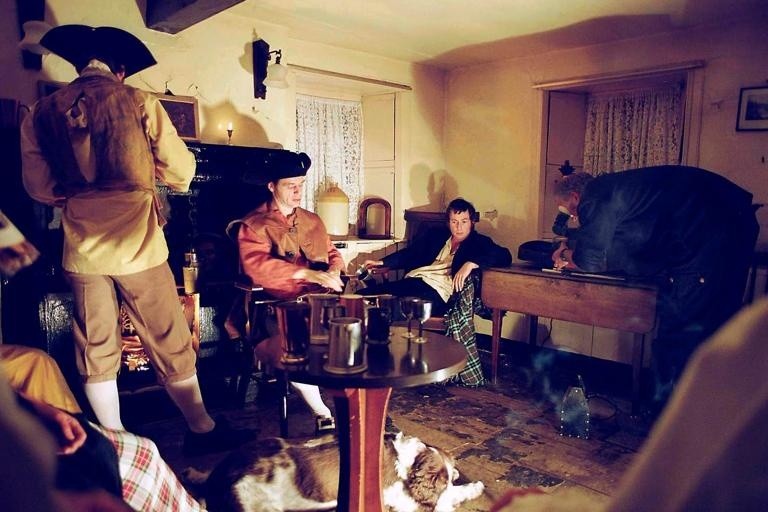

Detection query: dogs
(175, 428), (487, 512)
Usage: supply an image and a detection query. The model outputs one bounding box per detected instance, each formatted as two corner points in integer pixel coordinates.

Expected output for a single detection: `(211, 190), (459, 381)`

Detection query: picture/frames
(148, 92), (200, 142)
(732, 84), (768, 133)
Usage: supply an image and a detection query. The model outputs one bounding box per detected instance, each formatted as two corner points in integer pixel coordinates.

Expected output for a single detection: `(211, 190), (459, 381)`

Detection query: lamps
(250, 28), (288, 102)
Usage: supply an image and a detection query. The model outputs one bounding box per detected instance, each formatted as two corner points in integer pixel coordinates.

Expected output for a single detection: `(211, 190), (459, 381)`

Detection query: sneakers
(182, 415), (258, 459)
(614, 405), (658, 437)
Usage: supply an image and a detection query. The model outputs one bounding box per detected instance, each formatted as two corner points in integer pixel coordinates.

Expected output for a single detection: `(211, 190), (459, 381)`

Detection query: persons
(236, 151), (405, 438)
(0, 346), (208, 512)
(19, 25), (239, 453)
(352, 199), (513, 317)
(551, 165), (760, 430)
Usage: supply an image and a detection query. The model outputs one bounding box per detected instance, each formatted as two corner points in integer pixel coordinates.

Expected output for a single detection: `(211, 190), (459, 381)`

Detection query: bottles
(183, 252), (199, 295)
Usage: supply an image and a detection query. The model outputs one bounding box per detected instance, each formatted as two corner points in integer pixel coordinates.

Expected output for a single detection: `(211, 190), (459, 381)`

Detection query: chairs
(222, 272), (359, 438)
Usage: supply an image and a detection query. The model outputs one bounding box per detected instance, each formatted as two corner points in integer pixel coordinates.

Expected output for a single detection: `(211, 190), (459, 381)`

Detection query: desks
(478, 266), (661, 426)
(256, 326), (469, 511)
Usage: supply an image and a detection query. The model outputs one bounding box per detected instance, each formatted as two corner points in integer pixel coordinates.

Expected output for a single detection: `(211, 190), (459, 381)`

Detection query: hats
(39, 24), (157, 78)
(245, 149), (312, 188)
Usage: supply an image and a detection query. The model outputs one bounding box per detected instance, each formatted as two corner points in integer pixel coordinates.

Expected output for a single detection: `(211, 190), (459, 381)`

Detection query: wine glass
(377, 296), (432, 344)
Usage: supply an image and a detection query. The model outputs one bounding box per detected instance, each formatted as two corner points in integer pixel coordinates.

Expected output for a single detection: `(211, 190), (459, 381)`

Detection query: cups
(400, 343), (429, 374)
(275, 293), (390, 375)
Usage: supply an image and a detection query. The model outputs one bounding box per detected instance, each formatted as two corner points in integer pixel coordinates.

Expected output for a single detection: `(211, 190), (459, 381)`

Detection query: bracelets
(559, 248), (569, 263)
